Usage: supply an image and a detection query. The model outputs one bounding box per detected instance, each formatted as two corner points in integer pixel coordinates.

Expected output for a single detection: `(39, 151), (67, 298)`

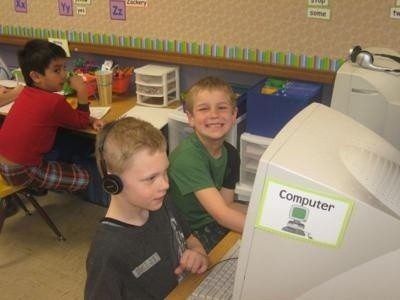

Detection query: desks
(0, 85), (183, 143)
(158, 229), (243, 300)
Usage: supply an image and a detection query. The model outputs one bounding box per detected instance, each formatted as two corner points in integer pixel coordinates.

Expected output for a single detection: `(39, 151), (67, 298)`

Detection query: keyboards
(190, 239), (241, 300)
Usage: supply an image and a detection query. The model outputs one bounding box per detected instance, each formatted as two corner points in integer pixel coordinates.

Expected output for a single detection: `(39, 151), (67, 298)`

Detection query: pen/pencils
(112, 64), (135, 77)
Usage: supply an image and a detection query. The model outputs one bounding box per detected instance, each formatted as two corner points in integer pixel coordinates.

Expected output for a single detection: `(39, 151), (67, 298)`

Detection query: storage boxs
(246, 77), (323, 139)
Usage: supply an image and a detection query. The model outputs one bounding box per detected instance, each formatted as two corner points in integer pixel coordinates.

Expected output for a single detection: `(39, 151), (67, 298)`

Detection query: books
(0, 100), (15, 117)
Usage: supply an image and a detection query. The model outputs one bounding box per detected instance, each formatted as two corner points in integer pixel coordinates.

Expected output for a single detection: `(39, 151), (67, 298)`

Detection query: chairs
(0, 174), (66, 246)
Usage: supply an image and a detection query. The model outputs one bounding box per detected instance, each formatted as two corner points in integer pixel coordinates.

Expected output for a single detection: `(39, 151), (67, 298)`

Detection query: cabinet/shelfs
(235, 133), (274, 202)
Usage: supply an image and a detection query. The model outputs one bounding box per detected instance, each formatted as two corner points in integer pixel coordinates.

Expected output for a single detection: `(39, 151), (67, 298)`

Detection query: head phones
(99, 118), (127, 195)
(349, 45), (400, 72)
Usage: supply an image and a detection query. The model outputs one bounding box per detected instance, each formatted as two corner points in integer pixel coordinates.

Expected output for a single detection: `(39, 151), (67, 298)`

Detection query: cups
(94, 70), (113, 106)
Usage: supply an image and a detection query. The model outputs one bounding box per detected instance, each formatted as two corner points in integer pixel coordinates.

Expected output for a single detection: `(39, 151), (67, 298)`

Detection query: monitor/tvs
(331, 47), (400, 152)
(232, 102), (400, 300)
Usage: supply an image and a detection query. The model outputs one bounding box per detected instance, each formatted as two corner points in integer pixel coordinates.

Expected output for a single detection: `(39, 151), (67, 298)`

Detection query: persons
(82, 75), (248, 300)
(0, 39), (107, 193)
